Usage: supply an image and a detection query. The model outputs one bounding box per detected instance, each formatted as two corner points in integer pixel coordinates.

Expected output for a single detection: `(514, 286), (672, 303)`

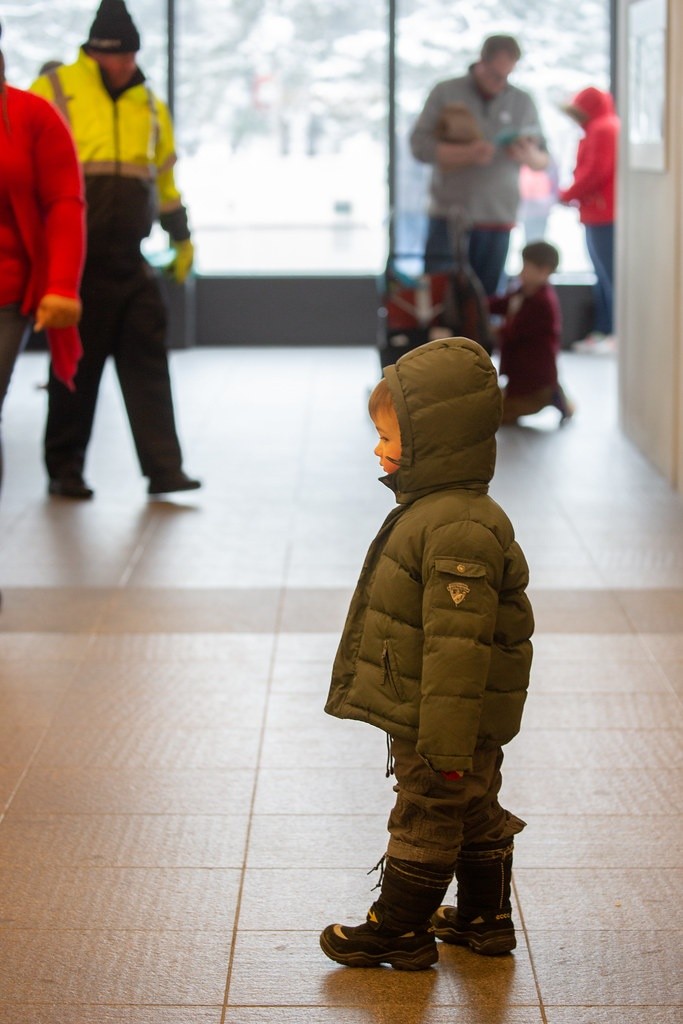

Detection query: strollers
(375, 249), (500, 375)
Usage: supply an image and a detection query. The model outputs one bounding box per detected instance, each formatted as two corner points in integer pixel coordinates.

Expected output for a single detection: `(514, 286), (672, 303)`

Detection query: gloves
(34, 296), (81, 333)
(163, 236), (195, 283)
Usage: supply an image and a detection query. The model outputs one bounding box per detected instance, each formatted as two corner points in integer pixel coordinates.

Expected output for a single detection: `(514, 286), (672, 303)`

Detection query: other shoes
(574, 333), (618, 355)
(559, 405), (574, 427)
(147, 476), (201, 494)
(48, 478), (92, 500)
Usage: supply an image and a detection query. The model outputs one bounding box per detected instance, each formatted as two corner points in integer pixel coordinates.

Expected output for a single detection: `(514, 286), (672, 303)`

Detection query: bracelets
(465, 143), (480, 167)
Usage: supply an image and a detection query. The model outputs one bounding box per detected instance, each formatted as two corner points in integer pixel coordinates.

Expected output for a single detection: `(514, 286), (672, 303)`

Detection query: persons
(405, 35), (550, 295)
(312, 336), (537, 970)
(560, 86), (621, 355)
(477, 242), (572, 426)
(0, 20), (87, 502)
(29, 0), (201, 499)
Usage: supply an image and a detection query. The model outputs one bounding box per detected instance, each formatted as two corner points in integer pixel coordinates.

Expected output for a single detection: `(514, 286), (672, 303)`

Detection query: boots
(320, 853), (454, 971)
(429, 836), (516, 955)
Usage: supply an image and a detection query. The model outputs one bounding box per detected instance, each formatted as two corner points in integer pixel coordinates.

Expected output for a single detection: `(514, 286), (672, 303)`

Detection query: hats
(81, 0), (141, 53)
